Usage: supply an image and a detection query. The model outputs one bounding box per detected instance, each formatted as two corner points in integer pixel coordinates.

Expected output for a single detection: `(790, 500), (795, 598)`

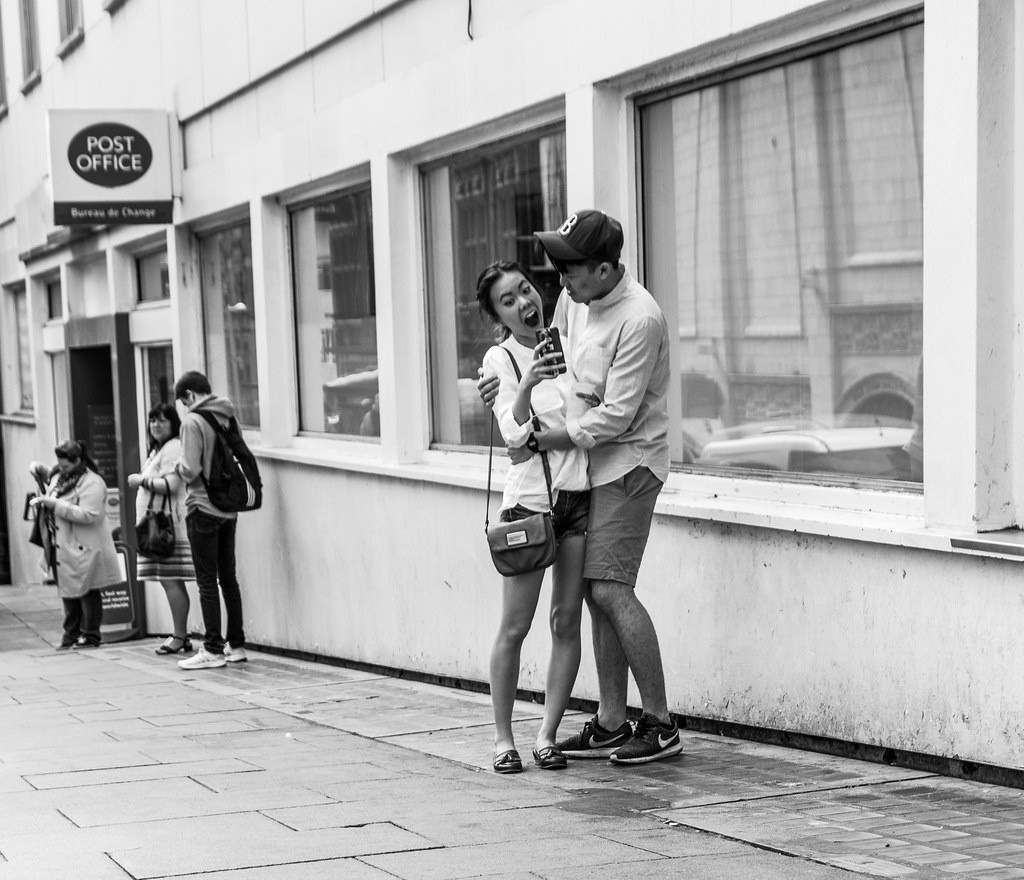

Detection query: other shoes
(55, 641), (95, 650)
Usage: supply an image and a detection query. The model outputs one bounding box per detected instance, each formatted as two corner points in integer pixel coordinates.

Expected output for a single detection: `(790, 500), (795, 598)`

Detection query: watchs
(527, 431), (540, 454)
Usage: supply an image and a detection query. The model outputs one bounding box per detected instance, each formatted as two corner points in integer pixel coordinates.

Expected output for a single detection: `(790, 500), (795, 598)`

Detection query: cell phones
(536, 326), (567, 375)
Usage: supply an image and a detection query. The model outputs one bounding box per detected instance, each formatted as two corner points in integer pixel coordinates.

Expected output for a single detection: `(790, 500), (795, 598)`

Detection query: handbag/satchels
(132, 478), (176, 558)
(488, 513), (558, 578)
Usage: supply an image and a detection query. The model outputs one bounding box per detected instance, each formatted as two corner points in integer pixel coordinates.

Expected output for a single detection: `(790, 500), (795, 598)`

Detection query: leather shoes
(533, 746), (567, 769)
(492, 750), (522, 773)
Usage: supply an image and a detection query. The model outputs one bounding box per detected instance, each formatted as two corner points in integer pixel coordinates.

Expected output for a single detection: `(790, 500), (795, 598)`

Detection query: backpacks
(191, 410), (262, 512)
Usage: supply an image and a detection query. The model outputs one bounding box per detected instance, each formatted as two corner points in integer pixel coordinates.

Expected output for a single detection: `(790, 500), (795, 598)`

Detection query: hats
(533, 209), (624, 261)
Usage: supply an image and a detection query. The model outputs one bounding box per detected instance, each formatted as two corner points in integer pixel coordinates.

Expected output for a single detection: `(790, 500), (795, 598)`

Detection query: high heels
(155, 635), (192, 654)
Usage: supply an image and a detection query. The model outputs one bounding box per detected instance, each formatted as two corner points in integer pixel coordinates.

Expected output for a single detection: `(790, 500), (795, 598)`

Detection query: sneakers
(223, 641), (247, 661)
(609, 714), (683, 764)
(554, 713), (633, 757)
(177, 648), (227, 668)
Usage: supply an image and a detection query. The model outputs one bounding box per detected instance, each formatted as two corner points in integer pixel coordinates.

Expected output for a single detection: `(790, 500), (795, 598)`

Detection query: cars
(697, 410), (923, 484)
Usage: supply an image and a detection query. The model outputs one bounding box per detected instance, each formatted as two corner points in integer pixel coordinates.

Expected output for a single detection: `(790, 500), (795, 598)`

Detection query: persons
(476, 210), (686, 768)
(30, 439), (124, 653)
(127, 402), (198, 655)
(474, 258), (595, 775)
(174, 371), (264, 670)
(360, 393), (381, 437)
